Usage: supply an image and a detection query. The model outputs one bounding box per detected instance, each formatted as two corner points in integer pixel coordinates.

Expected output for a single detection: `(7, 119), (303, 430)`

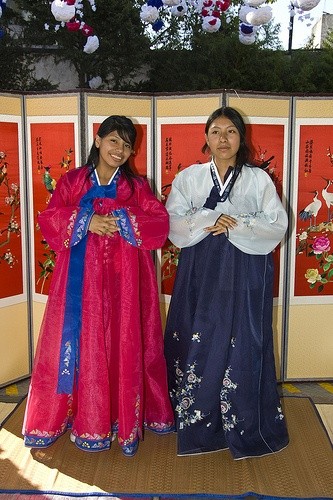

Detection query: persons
(22, 115), (176, 457)
(165, 106), (290, 461)
